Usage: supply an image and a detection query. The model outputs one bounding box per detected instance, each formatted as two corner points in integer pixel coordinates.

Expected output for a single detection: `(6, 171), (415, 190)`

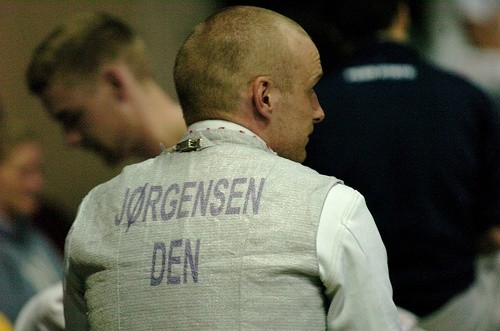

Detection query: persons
(62, 5), (409, 331)
(29, 10), (189, 166)
(1, 126), (70, 331)
(426, 0), (499, 98)
(302, 1), (500, 331)
